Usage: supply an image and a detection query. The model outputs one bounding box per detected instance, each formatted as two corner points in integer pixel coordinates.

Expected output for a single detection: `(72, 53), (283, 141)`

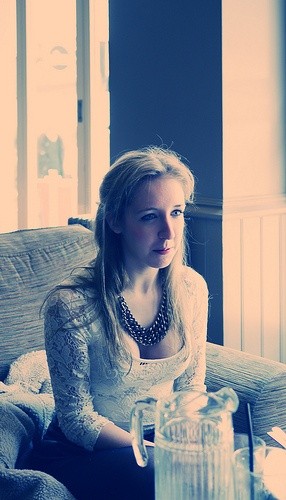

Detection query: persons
(37, 145), (208, 500)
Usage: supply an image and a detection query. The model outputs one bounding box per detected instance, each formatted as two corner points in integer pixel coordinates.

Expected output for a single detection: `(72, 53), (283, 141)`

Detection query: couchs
(1, 225), (285, 499)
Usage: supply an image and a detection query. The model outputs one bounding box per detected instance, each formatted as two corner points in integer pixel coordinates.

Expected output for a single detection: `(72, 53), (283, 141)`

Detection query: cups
(233, 433), (266, 500)
(232, 445), (286, 500)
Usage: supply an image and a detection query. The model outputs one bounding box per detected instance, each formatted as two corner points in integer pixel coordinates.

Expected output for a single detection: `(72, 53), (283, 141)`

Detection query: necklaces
(118, 287), (174, 346)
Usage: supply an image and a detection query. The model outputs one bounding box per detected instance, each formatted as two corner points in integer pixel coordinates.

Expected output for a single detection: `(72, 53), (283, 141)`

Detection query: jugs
(128, 387), (240, 500)
